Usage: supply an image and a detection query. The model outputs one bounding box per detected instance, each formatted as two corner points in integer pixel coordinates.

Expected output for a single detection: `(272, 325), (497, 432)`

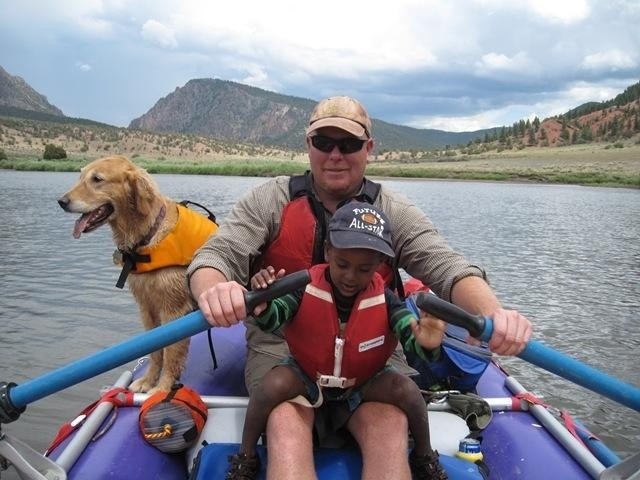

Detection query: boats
(41, 284), (629, 480)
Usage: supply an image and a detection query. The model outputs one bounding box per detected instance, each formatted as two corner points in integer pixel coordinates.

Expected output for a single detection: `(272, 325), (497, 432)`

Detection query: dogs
(55, 153), (220, 398)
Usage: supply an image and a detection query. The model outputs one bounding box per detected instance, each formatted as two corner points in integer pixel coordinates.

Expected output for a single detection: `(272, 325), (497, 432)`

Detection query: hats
(308, 94), (371, 140)
(329, 201), (397, 259)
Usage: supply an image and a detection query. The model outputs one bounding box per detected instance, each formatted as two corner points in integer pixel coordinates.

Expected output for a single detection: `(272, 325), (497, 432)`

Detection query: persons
(224, 202), (452, 480)
(185, 95), (533, 480)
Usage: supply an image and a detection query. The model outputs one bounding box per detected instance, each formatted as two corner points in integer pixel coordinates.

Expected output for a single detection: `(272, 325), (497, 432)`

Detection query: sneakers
(409, 449), (448, 479)
(225, 453), (260, 479)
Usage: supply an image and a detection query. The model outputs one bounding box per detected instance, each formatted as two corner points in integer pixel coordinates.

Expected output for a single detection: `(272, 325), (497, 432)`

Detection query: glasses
(311, 132), (365, 154)
